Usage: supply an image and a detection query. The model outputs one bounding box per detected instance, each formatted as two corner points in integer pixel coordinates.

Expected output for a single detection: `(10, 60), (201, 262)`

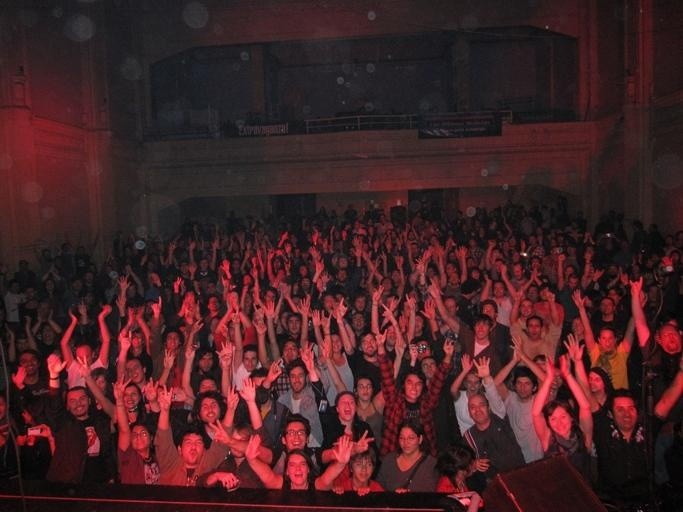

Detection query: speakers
(482, 455), (608, 511)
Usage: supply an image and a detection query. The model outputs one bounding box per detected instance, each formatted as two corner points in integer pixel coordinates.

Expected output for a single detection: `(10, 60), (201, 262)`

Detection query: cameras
(415, 344), (426, 352)
(318, 400), (328, 412)
(445, 328), (458, 340)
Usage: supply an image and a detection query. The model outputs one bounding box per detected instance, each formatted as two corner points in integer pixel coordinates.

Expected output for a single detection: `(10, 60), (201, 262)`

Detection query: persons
(0, 193), (681, 512)
